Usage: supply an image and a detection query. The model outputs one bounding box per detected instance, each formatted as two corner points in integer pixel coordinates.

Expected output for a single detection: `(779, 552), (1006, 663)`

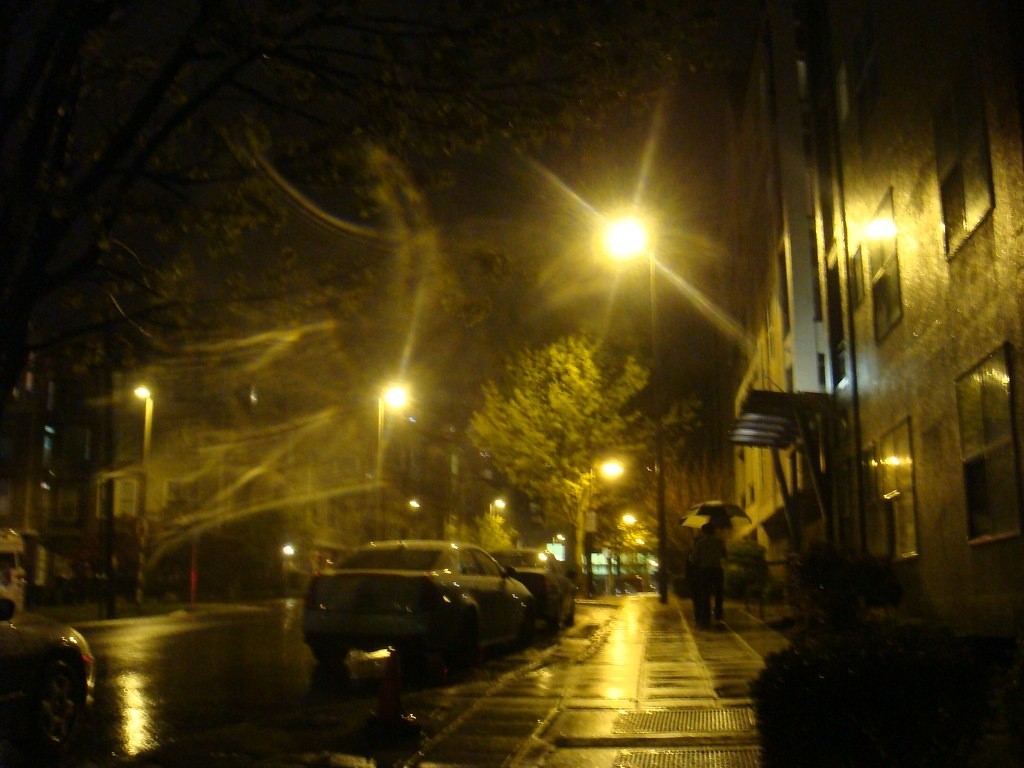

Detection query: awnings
(728, 390), (830, 448)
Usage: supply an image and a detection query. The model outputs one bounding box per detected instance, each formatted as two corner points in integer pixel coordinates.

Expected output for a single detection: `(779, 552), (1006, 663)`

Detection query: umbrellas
(680, 500), (752, 530)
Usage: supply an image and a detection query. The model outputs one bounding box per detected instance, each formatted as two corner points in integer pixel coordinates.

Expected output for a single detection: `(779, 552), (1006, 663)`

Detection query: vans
(0, 528), (29, 617)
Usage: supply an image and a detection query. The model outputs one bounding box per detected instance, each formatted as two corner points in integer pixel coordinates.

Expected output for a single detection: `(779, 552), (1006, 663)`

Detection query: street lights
(127, 380), (154, 605)
(367, 382), (409, 542)
(605, 208), (672, 605)
(281, 543), (296, 601)
(579, 453), (626, 597)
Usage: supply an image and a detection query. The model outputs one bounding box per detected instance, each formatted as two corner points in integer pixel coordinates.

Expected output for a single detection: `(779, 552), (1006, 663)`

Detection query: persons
(682, 522), (728, 627)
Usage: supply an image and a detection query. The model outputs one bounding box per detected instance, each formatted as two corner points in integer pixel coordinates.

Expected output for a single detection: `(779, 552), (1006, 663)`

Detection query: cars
(0, 595), (96, 747)
(485, 546), (579, 638)
(304, 540), (540, 690)
